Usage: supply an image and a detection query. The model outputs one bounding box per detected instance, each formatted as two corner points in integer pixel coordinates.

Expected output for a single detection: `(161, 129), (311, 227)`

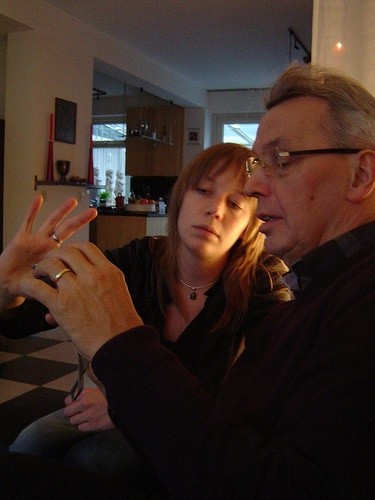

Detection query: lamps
(294, 39), (299, 50)
(303, 56), (311, 63)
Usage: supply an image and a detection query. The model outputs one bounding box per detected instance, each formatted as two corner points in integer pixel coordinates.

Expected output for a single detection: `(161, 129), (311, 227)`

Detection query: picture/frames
(53, 97), (77, 145)
(185, 128), (201, 145)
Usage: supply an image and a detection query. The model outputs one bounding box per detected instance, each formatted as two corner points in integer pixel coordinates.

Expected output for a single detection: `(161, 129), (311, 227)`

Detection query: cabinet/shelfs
(127, 106), (184, 176)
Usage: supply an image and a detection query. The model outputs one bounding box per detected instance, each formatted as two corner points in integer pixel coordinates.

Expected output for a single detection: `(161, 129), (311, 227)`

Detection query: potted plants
(100, 191), (109, 204)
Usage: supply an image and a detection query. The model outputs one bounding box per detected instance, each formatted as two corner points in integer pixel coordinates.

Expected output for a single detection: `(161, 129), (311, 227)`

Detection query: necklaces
(176, 273), (218, 300)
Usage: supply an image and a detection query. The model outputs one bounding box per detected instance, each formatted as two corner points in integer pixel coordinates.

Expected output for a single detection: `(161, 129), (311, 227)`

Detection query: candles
(50, 113), (53, 140)
(90, 129), (93, 149)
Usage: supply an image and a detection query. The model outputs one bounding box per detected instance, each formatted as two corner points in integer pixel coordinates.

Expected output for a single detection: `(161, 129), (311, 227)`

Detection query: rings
(55, 268), (72, 280)
(53, 234), (62, 244)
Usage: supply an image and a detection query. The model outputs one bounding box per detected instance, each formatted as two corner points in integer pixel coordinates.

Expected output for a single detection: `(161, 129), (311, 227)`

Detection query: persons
(0, 143), (295, 479)
(0, 61), (375, 500)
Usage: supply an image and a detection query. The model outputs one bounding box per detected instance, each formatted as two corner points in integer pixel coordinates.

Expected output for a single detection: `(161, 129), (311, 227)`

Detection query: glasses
(245, 147), (362, 177)
